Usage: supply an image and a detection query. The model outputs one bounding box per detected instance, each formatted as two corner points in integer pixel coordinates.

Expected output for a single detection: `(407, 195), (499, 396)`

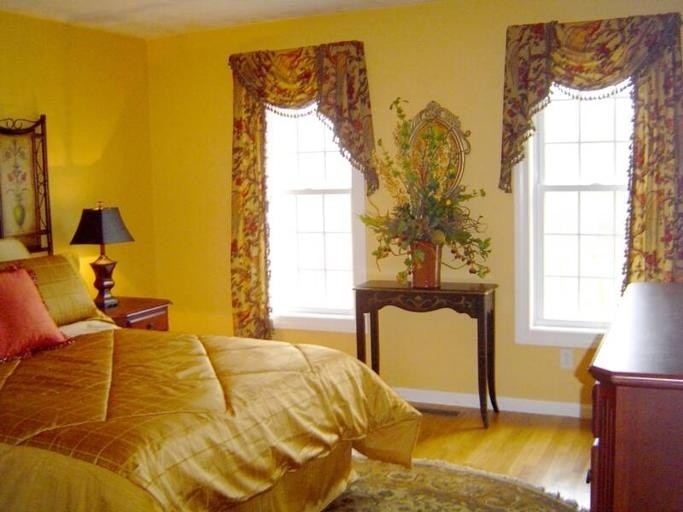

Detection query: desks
(354, 278), (503, 432)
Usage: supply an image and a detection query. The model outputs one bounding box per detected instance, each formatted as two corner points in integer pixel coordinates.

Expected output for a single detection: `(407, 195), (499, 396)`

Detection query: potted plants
(350, 96), (495, 289)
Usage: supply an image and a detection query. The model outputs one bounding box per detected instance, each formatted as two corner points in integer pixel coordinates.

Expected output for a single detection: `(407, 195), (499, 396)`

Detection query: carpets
(312, 450), (592, 512)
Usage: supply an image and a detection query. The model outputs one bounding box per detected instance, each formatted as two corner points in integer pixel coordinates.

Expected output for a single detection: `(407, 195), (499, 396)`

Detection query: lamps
(68, 200), (137, 309)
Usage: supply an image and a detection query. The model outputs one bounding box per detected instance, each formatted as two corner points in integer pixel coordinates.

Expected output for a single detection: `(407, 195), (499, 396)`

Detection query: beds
(1, 236), (425, 512)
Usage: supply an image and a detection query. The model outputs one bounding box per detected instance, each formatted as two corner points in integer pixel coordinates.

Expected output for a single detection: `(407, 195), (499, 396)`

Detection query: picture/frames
(400, 99), (474, 203)
(0, 112), (54, 259)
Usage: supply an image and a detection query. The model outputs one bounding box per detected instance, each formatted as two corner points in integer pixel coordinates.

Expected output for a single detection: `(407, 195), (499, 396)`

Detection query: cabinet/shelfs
(585, 280), (683, 512)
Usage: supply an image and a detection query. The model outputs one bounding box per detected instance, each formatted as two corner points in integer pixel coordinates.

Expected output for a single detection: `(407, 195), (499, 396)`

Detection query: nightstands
(94, 296), (176, 331)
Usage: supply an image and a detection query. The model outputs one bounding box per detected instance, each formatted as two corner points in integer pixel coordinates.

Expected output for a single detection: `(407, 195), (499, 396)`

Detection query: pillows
(1, 264), (75, 363)
(0, 254), (117, 328)
